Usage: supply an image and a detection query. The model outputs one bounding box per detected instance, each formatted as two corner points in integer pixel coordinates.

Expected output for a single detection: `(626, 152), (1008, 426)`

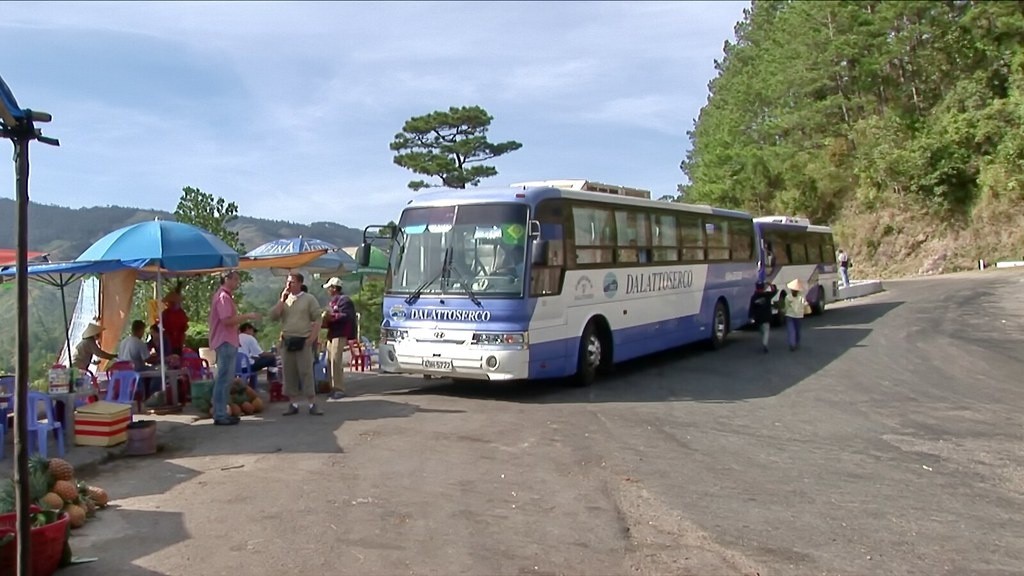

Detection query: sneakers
(214, 415), (240, 425)
(310, 404), (325, 414)
(282, 403), (300, 416)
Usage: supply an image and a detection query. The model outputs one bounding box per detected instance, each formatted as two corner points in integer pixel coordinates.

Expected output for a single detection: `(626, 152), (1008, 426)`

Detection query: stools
(46, 391), (100, 449)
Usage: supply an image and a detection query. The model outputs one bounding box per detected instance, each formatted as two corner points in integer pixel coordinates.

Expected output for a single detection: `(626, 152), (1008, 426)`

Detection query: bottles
(49, 364), (69, 394)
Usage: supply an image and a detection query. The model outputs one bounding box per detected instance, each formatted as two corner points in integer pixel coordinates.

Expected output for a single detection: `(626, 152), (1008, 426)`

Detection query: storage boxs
(73, 400), (132, 447)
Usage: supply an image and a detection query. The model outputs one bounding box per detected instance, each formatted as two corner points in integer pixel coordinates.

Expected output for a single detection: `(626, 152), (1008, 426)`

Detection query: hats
(323, 277), (343, 289)
(82, 323), (104, 338)
(756, 280), (764, 289)
(162, 290), (182, 303)
(240, 323), (258, 333)
(787, 279), (803, 292)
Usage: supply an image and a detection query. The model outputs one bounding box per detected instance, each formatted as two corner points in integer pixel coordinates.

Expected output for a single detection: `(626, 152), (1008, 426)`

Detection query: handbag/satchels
(803, 305), (812, 314)
(281, 335), (308, 352)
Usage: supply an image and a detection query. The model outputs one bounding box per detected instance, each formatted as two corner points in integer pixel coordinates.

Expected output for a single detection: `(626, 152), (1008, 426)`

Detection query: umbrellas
(73, 217), (239, 395)
(342, 244), (389, 304)
(304, 245), (358, 274)
(239, 235), (339, 275)
(0, 259), (133, 368)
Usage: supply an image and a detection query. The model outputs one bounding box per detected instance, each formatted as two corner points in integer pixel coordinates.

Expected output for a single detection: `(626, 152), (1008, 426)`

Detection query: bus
(706, 215), (840, 330)
(360, 178), (761, 389)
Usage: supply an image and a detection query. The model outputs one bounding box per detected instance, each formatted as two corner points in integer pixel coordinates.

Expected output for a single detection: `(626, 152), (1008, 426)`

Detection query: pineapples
(28, 453), (107, 527)
(208, 376), (263, 417)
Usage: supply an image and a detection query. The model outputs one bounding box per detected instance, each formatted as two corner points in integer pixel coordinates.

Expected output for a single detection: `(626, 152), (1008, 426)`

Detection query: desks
(97, 370), (193, 406)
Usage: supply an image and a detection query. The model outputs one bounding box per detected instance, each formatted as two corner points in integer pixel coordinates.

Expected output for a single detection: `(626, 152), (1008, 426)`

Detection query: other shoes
(327, 388), (345, 399)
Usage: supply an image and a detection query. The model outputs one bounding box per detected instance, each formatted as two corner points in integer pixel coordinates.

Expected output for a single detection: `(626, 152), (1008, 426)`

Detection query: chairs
(348, 335), (382, 372)
(0, 341), (329, 461)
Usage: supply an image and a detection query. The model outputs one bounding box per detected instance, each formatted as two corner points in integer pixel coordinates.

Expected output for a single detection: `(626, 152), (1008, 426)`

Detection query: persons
(238, 323), (276, 388)
(321, 277), (355, 398)
(510, 244), (524, 281)
(269, 272), (324, 416)
(783, 279), (808, 351)
(209, 271), (262, 425)
(839, 249), (850, 286)
(73, 290), (189, 400)
(749, 281), (778, 352)
